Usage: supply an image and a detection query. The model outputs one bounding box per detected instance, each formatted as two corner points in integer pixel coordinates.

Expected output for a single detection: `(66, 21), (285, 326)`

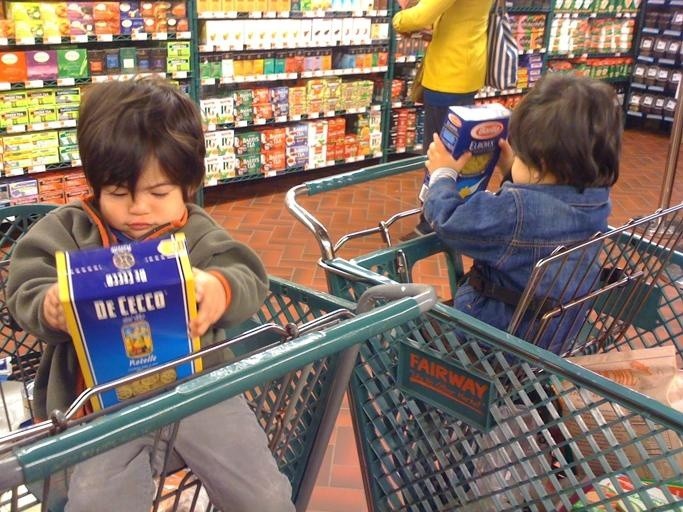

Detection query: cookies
(116, 369), (177, 400)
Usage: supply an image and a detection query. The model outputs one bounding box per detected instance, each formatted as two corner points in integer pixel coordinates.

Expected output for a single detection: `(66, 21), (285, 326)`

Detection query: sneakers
(398, 231), (419, 243)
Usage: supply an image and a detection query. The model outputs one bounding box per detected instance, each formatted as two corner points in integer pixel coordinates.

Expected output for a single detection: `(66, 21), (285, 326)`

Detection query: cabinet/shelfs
(625, 5), (683, 133)
(0, 2), (194, 232)
(193, 0), (393, 198)
(388, 2), (557, 165)
(542, 2), (646, 133)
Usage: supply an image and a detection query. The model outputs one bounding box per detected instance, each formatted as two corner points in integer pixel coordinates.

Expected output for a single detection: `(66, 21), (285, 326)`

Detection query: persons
(1, 75), (299, 512)
(386, 69), (625, 512)
(389, 0), (496, 160)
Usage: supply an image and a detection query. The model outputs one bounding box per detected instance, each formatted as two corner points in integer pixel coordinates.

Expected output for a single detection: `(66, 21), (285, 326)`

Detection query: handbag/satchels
(412, 57), (426, 103)
(486, 11), (518, 91)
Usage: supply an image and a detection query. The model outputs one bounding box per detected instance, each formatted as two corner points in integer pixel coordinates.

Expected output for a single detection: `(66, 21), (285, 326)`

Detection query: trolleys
(0, 154), (683, 510)
